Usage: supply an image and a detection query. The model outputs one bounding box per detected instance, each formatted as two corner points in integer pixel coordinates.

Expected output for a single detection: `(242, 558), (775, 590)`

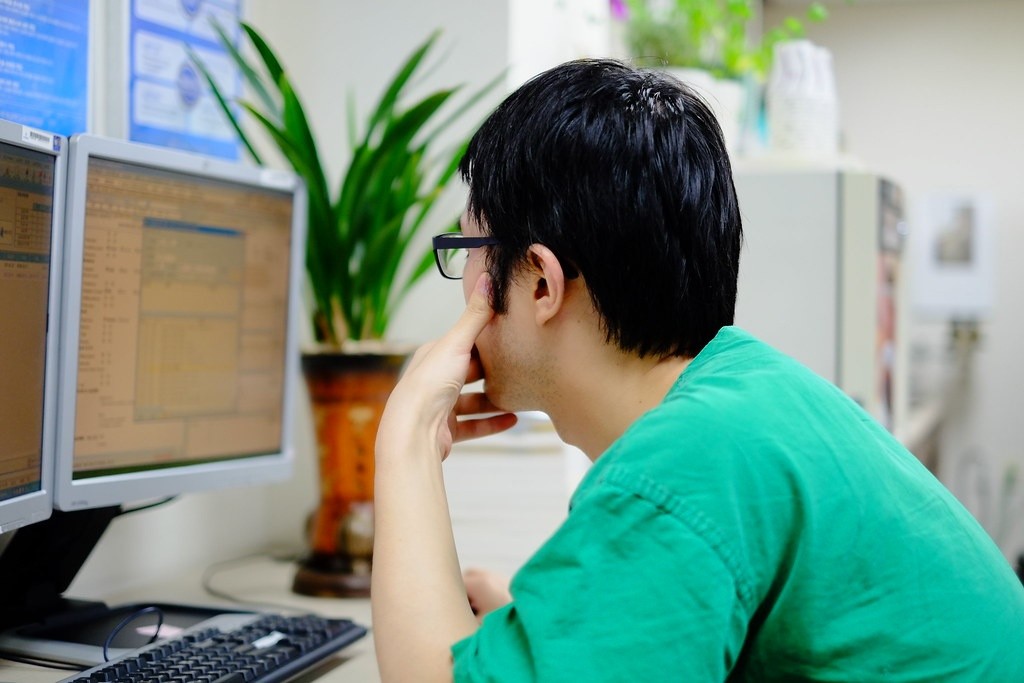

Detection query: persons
(371, 58), (1024, 683)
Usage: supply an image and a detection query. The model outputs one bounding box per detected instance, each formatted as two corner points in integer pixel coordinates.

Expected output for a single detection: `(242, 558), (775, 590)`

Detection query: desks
(0, 563), (386, 683)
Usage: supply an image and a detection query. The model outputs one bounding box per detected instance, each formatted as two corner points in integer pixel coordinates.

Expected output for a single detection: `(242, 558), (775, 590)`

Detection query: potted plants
(627, 0), (826, 147)
(185, 19), (509, 599)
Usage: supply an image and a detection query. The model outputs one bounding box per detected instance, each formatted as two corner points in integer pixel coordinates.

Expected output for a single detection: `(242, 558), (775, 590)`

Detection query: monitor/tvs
(0, 111), (310, 668)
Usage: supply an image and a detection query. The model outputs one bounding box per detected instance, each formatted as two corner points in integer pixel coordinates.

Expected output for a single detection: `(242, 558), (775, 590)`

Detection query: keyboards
(56, 614), (368, 683)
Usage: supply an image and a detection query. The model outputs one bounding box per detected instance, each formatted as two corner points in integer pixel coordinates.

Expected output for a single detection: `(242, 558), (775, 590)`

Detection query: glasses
(432, 232), (578, 281)
